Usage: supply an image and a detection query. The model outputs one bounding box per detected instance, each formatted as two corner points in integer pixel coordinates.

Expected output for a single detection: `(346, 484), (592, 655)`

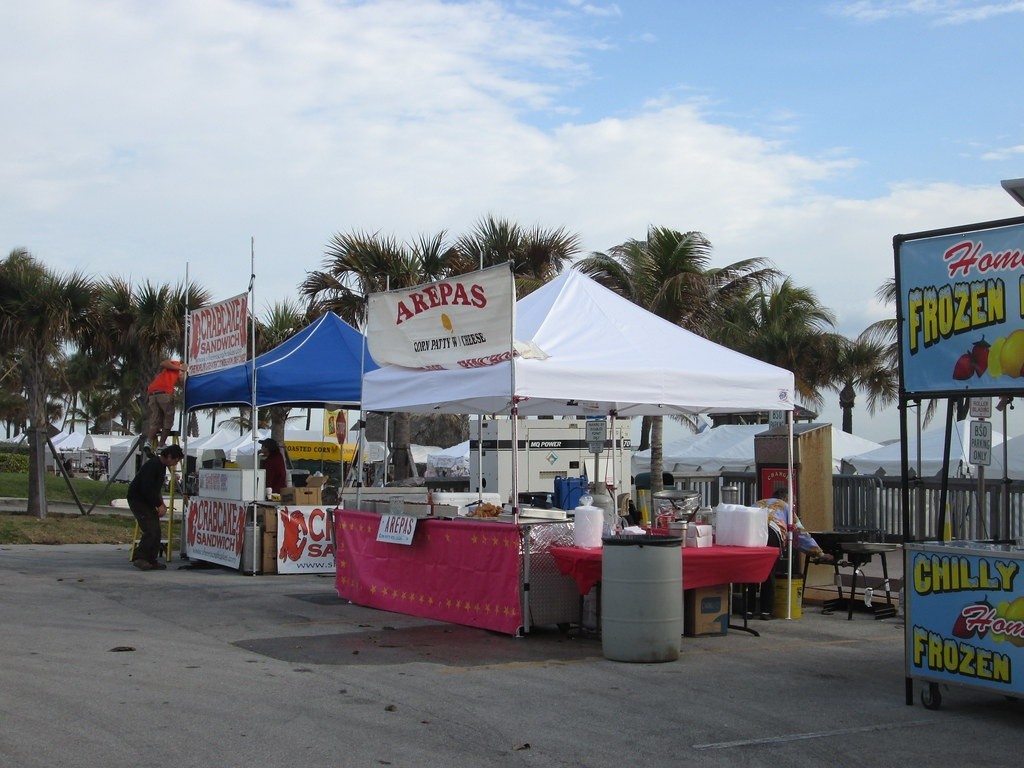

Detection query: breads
(477, 503), (503, 517)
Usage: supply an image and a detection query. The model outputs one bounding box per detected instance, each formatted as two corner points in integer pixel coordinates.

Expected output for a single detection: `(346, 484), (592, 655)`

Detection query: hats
(258, 438), (276, 452)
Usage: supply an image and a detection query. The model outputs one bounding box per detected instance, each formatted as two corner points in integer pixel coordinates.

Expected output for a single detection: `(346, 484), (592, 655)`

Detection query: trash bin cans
(601, 535), (682, 664)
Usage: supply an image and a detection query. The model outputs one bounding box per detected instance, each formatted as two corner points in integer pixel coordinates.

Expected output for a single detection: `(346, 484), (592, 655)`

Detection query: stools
(135, 538), (168, 558)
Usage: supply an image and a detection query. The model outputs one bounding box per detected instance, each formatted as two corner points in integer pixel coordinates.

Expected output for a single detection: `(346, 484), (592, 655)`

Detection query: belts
(149, 391), (168, 396)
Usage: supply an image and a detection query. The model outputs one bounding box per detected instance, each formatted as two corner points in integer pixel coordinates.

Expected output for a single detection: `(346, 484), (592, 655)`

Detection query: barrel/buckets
(668, 521), (695, 548)
(600, 533), (684, 662)
(773, 570), (803, 619)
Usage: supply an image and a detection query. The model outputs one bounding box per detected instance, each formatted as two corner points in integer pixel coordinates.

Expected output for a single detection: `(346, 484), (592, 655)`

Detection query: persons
(321, 471), (339, 504)
(126, 444), (185, 571)
(143, 360), (188, 459)
(746, 488), (833, 621)
(258, 438), (286, 493)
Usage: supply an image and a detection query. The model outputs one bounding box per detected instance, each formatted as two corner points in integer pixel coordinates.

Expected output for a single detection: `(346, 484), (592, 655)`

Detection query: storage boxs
(685, 586), (730, 635)
(280, 476), (326, 507)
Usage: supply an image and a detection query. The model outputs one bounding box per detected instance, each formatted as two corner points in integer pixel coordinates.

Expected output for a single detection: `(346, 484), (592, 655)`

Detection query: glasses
(793, 504), (795, 507)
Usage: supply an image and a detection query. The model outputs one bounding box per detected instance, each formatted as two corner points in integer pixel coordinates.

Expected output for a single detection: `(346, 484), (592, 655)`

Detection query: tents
(840, 419), (1011, 538)
(630, 424), (885, 505)
(425, 412), (710, 493)
(356, 266), (795, 619)
(971, 434), (1024, 540)
(180, 310), (395, 582)
(6, 407), (443, 486)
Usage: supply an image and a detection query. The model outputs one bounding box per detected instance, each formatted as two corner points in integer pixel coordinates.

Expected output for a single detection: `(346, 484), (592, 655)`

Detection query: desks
(187, 475), (199, 495)
(550, 543), (780, 636)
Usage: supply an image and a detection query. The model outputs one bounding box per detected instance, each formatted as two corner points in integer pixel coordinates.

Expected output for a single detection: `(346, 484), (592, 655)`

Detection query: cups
(266, 487), (272, 500)
(389, 496), (404, 515)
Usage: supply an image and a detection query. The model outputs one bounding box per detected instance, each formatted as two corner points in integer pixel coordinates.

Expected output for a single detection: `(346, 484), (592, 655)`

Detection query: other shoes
(760, 610), (777, 619)
(157, 444), (168, 454)
(144, 442), (154, 458)
(744, 611), (752, 619)
(151, 560), (166, 569)
(133, 558), (153, 570)
